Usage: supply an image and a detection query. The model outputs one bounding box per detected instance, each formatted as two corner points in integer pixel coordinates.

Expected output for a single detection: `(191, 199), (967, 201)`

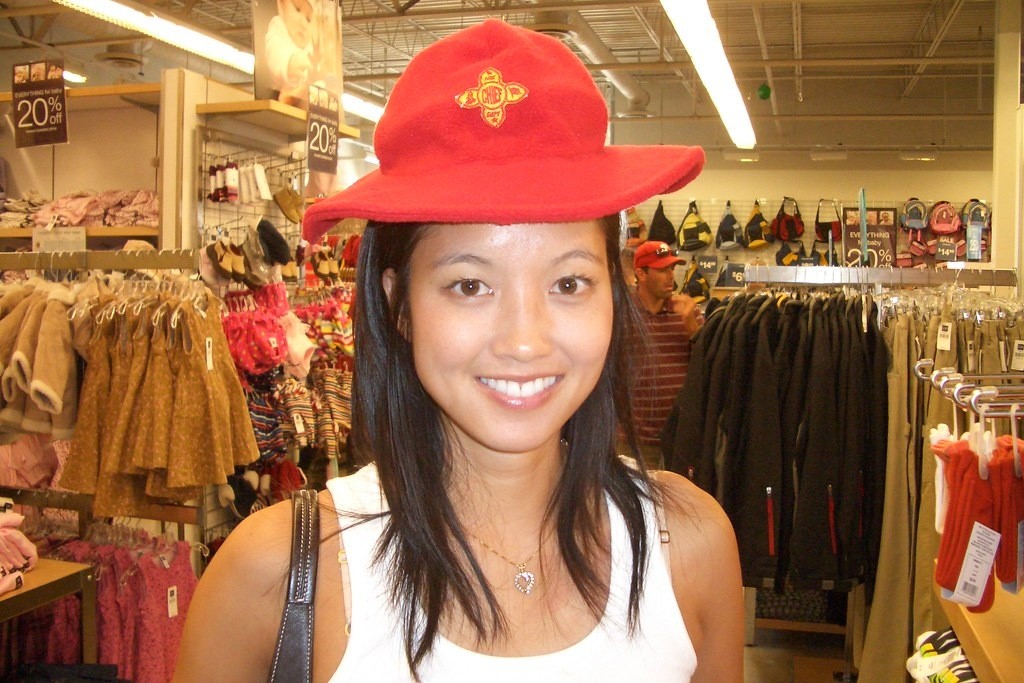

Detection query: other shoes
(198, 186), (339, 285)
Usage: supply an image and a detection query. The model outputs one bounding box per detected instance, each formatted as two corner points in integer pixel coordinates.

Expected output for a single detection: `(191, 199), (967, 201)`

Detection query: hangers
(11, 247), (230, 330)
(16, 488), (209, 587)
(726, 264), (1024, 327)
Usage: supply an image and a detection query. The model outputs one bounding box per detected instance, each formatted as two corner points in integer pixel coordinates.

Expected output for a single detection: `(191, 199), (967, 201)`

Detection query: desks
(931, 556), (1024, 683)
(0, 560), (97, 677)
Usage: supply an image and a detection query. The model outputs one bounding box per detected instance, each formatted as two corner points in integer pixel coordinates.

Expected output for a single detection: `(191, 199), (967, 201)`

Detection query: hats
(633, 241), (687, 269)
(301, 17), (705, 244)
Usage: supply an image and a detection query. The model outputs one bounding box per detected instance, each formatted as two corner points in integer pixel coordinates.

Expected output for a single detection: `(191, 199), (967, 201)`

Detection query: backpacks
(901, 197), (929, 231)
(929, 200), (962, 236)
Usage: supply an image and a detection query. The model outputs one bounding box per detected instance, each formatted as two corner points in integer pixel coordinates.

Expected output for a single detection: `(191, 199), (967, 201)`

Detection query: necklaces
(458, 438), (569, 593)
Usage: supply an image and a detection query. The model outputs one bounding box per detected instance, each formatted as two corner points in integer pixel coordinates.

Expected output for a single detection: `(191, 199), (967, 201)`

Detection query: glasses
(633, 248), (679, 265)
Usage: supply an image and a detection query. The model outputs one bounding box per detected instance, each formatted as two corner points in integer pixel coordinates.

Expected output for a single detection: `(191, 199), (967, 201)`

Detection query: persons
(613, 242), (702, 473)
(265, 0), (330, 104)
(172, 18), (745, 683)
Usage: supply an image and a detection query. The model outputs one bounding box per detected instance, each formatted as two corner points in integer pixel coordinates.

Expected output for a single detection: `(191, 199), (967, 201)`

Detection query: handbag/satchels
(776, 239), (808, 266)
(680, 255), (710, 304)
(814, 199), (842, 242)
(676, 202), (712, 251)
(625, 205), (648, 249)
(962, 199), (989, 229)
(772, 197), (806, 242)
(648, 200), (676, 245)
(716, 202), (743, 250)
(810, 238), (839, 267)
(745, 202), (776, 251)
(715, 257), (746, 288)
